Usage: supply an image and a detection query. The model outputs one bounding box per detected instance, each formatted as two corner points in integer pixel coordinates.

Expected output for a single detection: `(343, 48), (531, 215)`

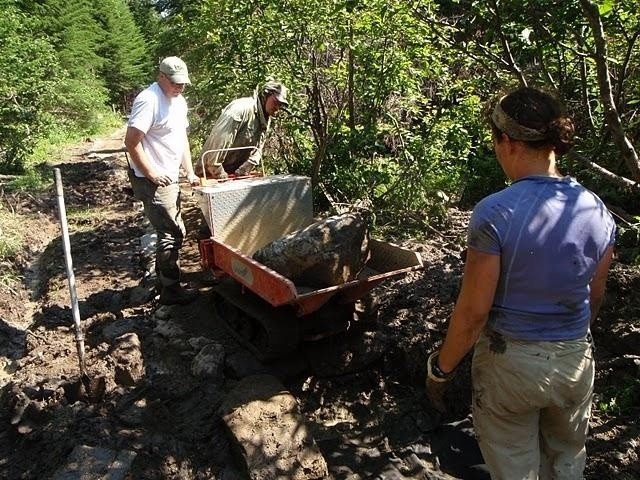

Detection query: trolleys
(198, 146), (427, 359)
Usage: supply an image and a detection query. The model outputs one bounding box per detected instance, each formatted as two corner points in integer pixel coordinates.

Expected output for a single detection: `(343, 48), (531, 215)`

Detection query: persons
(124, 56), (202, 307)
(423, 85), (618, 479)
(193, 80), (292, 286)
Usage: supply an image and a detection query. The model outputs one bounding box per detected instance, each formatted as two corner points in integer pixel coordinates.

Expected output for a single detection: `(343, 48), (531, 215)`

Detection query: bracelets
(431, 357), (459, 378)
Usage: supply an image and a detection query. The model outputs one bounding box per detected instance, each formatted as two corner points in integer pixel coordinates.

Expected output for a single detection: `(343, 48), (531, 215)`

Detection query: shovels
(54, 168), (105, 405)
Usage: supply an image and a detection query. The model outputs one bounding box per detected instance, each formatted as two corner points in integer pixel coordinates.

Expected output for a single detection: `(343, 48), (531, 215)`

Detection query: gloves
(423, 350), (452, 411)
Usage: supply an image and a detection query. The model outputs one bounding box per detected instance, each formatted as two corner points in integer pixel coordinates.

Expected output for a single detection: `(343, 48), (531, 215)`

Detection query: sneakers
(159, 286), (198, 304)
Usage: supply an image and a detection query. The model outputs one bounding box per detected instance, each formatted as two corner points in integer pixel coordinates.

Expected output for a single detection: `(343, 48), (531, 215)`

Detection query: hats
(263, 81), (290, 107)
(160, 56), (191, 85)
